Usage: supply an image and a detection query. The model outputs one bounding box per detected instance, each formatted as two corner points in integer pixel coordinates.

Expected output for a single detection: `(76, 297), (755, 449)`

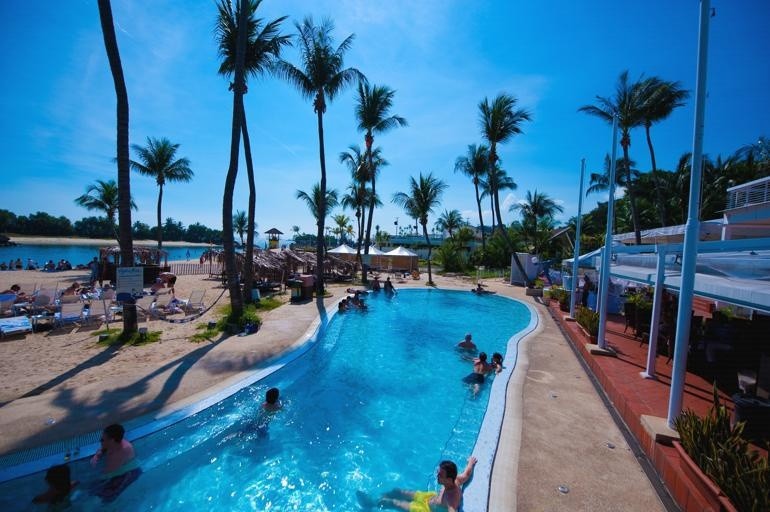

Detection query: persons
(338, 274), (398, 311)
(478, 284), (483, 294)
(456, 333), (476, 354)
(485, 350), (504, 374)
(90, 423), (137, 505)
(472, 288), (498, 294)
(359, 455), (478, 512)
(264, 387), (287, 409)
(583, 274), (591, 308)
(0, 256), (185, 324)
(464, 351), (488, 397)
(32, 461), (79, 502)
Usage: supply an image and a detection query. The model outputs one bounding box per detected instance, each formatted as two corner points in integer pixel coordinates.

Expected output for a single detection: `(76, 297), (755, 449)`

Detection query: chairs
(623, 302), (770, 450)
(0, 286), (206, 341)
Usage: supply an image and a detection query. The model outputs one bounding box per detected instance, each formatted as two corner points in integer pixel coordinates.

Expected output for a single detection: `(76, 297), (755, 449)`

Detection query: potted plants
(542, 284), (599, 344)
